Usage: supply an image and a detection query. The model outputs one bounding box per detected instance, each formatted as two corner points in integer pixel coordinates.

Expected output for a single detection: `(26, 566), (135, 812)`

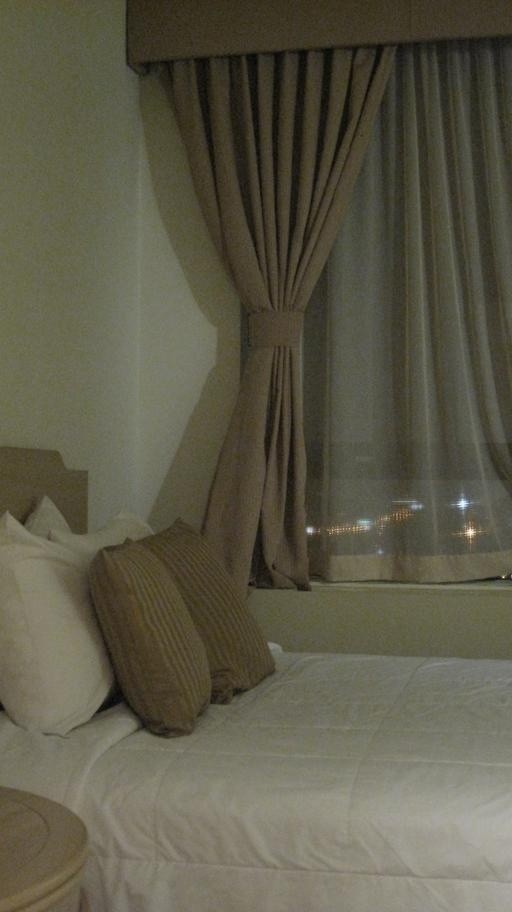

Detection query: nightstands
(0, 787), (92, 911)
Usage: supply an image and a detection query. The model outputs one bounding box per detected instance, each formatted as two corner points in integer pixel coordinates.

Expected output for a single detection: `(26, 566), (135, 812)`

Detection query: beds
(0, 444), (511, 911)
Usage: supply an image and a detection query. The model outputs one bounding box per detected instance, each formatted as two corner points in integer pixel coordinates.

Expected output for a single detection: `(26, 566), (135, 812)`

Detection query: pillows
(46, 514), (157, 556)
(136, 512), (278, 708)
(1, 491), (117, 739)
(87, 536), (213, 740)
